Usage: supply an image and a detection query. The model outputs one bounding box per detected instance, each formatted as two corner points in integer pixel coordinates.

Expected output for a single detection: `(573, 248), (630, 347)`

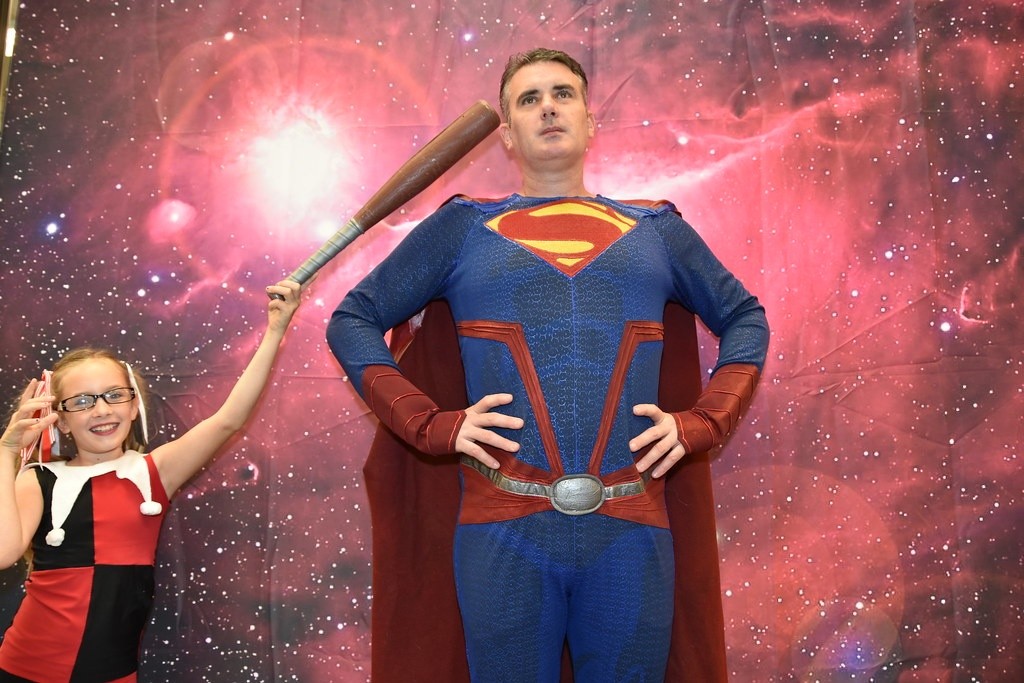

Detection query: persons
(326, 47), (771, 683)
(0, 277), (301, 683)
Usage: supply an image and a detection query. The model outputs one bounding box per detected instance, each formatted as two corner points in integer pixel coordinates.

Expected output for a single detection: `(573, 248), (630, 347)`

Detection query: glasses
(52, 387), (135, 413)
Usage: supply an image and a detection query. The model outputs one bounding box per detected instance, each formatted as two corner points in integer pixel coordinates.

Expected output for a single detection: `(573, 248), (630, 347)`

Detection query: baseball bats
(266, 99), (501, 300)
